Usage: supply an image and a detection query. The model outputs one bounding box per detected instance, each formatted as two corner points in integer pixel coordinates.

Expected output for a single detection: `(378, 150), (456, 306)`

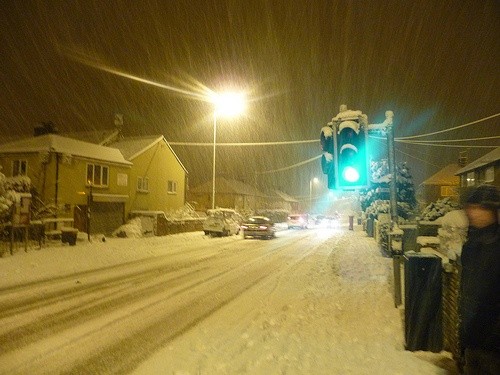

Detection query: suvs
(202, 208), (241, 236)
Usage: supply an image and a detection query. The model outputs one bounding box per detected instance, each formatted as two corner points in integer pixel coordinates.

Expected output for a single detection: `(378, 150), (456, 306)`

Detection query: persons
(458, 186), (500, 375)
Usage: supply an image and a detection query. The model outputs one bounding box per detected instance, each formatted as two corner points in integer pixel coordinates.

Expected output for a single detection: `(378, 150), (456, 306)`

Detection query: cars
(313, 215), (326, 225)
(241, 215), (277, 240)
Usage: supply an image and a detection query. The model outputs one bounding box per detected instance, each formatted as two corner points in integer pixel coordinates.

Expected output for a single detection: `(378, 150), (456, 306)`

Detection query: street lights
(211, 92), (245, 208)
(308, 177), (319, 204)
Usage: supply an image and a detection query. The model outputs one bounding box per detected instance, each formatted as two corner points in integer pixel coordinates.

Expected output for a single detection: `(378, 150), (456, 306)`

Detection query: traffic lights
(332, 114), (371, 190)
(319, 122), (336, 190)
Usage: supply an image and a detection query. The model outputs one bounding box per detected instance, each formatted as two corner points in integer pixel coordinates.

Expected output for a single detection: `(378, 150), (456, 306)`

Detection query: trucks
(287, 214), (308, 229)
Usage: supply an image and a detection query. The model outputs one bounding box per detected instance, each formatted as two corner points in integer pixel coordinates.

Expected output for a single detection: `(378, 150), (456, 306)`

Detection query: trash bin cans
(358, 218), (362, 225)
(405, 252), (442, 351)
(60, 226), (78, 245)
(366, 219), (373, 237)
